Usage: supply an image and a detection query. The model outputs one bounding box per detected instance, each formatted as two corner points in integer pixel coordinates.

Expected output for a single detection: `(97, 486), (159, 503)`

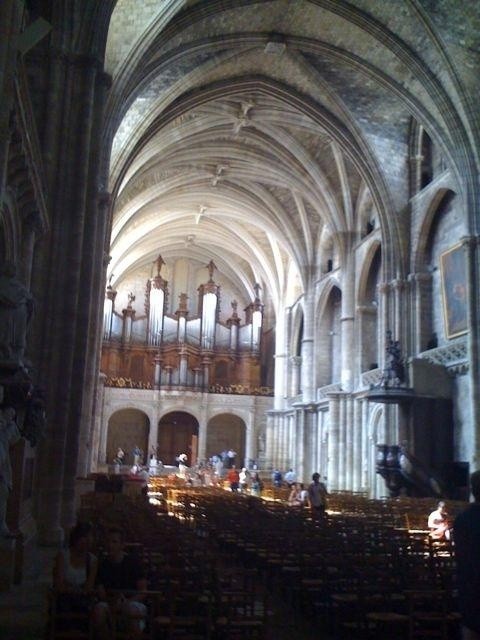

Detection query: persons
(428, 470), (480, 640)
(112, 444), (331, 512)
(49, 521), (148, 637)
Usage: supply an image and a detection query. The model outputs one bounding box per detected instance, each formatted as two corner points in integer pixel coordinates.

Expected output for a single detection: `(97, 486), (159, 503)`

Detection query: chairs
(76, 470), (477, 637)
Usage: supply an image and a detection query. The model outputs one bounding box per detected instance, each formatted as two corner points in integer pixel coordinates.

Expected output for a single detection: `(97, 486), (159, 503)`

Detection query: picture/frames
(438, 241), (471, 339)
